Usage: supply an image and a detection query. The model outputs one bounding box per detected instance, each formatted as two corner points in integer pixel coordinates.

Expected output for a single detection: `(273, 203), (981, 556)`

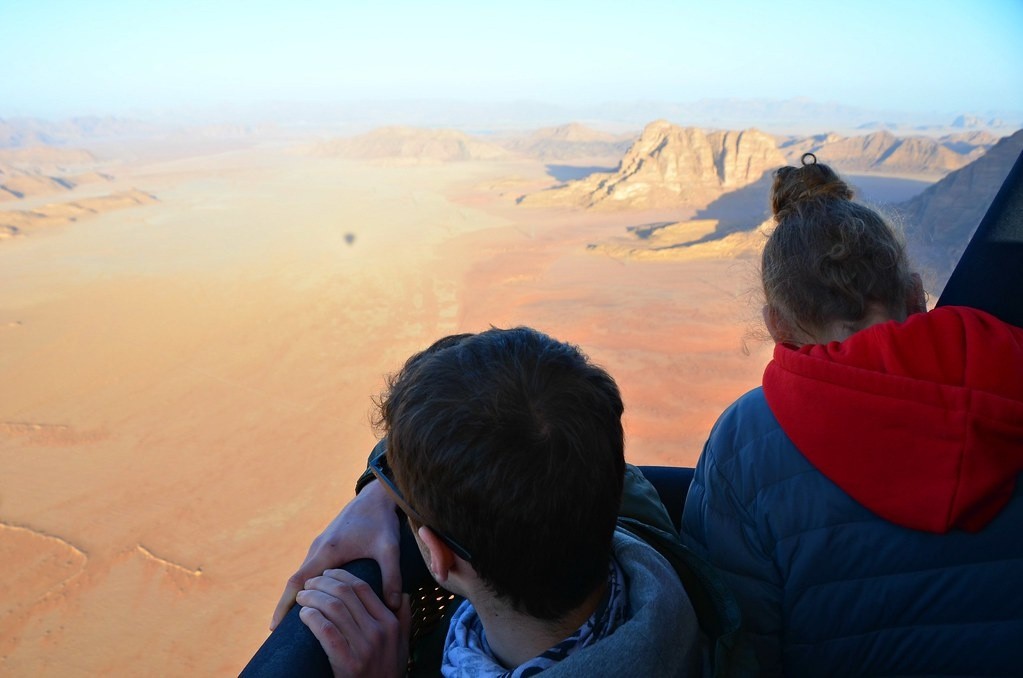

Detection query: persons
(269, 322), (706, 678)
(685, 151), (1022, 678)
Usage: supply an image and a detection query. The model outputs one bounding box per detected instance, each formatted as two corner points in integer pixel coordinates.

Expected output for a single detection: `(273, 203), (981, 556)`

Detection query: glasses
(364, 445), (473, 568)
